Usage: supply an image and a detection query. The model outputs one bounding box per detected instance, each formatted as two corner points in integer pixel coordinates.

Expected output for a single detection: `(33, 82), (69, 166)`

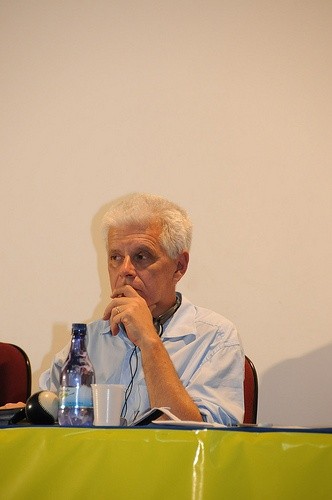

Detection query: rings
(114, 307), (120, 314)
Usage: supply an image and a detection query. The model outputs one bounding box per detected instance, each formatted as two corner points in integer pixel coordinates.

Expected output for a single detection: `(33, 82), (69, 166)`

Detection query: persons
(0, 193), (244, 427)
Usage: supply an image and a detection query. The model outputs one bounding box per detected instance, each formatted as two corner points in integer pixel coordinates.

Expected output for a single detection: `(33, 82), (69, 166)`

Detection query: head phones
(120, 295), (180, 338)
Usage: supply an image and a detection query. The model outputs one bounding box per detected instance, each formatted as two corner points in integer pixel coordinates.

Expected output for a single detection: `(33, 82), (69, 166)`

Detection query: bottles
(58, 324), (95, 427)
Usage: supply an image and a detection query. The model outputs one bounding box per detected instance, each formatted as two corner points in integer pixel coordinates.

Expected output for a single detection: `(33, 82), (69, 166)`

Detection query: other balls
(25, 390), (59, 425)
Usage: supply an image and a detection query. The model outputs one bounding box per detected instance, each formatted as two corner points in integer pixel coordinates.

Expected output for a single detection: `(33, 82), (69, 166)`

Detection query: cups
(89, 384), (125, 427)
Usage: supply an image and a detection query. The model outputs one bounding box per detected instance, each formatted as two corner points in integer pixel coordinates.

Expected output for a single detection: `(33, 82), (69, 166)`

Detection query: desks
(0, 425), (332, 500)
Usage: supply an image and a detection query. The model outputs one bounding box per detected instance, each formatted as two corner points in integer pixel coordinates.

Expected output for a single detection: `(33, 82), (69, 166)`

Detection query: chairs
(0, 342), (32, 404)
